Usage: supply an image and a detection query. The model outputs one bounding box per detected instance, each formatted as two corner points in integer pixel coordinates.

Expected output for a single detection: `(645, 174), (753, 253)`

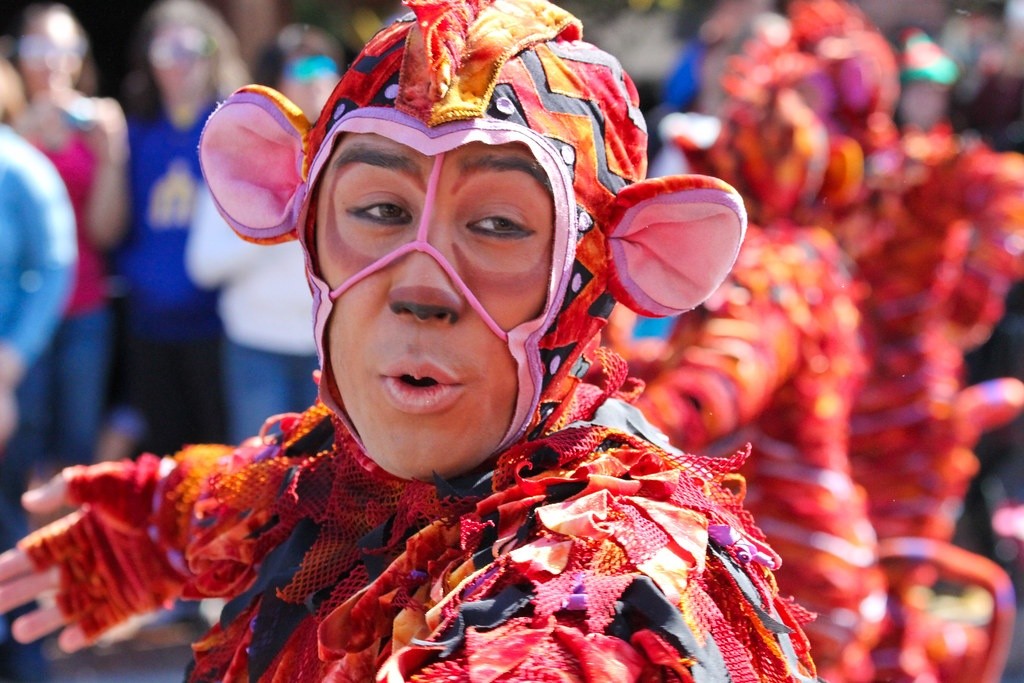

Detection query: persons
(0, 0), (821, 683)
(0, 2), (345, 683)
(628, 0), (1023, 683)
